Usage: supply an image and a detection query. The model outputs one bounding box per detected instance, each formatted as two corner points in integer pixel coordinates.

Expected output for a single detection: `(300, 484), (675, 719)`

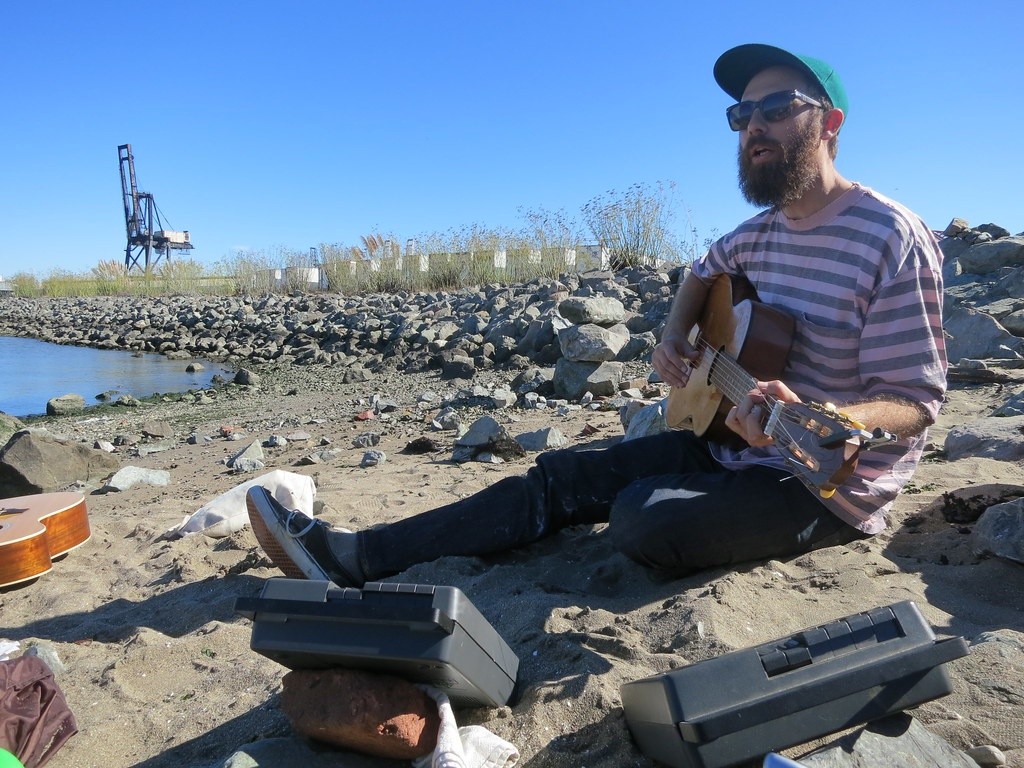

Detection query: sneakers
(246, 485), (360, 594)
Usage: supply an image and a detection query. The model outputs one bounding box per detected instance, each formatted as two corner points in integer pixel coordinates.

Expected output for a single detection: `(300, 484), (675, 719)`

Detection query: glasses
(724, 86), (827, 132)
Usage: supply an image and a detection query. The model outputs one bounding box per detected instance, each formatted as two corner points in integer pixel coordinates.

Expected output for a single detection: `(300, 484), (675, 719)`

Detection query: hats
(713, 44), (851, 134)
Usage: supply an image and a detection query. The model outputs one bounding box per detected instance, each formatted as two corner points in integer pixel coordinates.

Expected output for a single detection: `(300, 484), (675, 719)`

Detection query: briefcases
(250, 575), (525, 710)
(619, 596), (971, 768)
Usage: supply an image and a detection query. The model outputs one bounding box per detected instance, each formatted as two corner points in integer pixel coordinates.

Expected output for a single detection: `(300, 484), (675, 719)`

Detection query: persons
(245, 43), (948, 589)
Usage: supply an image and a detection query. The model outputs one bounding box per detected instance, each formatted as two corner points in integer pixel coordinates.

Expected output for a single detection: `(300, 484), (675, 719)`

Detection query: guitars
(664, 273), (864, 500)
(0, 490), (94, 591)
